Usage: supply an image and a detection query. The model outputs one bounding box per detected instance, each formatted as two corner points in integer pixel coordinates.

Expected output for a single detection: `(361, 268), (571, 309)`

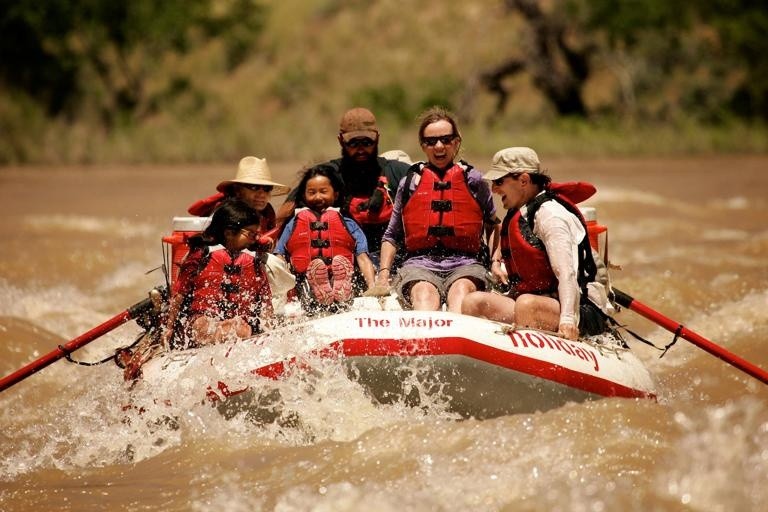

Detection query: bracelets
(492, 259), (501, 263)
(378, 268), (392, 274)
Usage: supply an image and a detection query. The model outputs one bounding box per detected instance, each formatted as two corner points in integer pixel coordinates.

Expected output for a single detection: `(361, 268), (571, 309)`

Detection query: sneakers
(305, 255), (353, 307)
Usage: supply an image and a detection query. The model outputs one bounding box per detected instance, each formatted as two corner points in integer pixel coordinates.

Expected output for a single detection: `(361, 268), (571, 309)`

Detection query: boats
(122, 310), (657, 426)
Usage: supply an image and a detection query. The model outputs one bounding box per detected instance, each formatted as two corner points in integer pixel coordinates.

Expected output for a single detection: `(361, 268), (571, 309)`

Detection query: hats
(215, 154), (291, 198)
(481, 146), (540, 180)
(339, 107), (379, 142)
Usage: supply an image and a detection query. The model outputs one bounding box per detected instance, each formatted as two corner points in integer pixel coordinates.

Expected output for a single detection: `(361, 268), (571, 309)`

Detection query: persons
(274, 161), (375, 317)
(364, 104), (508, 318)
(186, 154), (280, 264)
(273, 105), (419, 279)
(461, 146), (617, 341)
(159, 195), (276, 353)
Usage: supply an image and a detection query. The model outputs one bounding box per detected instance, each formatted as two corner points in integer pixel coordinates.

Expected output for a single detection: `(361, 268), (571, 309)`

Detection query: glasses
(490, 179), (508, 185)
(346, 137), (375, 147)
(424, 134), (456, 145)
(241, 182), (274, 193)
